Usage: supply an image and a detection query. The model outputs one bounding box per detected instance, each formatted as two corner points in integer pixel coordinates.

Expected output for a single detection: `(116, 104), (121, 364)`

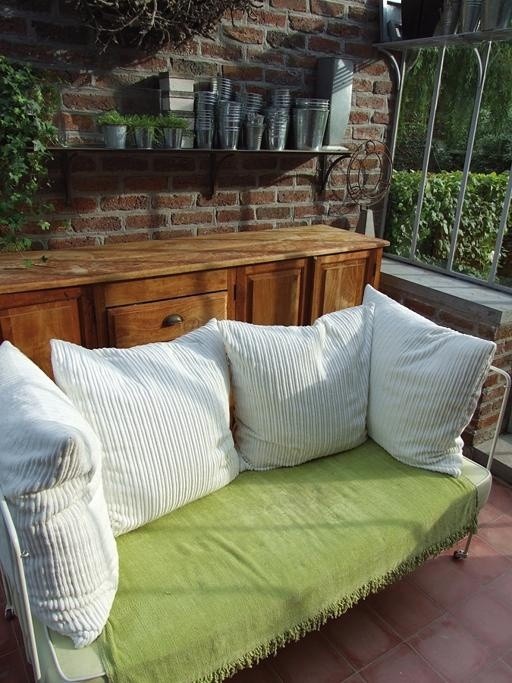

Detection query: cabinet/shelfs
(0, 224), (391, 378)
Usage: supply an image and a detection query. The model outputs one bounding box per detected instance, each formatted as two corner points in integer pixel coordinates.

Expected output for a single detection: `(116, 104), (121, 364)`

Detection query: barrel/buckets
(103, 75), (330, 150)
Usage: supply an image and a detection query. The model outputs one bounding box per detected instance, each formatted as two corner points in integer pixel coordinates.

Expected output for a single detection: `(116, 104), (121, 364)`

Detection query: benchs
(1, 366), (511, 683)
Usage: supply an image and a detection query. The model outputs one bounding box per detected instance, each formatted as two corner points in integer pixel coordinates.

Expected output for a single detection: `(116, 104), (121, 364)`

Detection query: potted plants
(99, 108), (188, 149)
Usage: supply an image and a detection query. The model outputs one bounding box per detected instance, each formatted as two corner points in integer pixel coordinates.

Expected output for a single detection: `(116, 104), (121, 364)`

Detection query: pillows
(51, 312), (238, 536)
(216, 299), (371, 471)
(0, 338), (119, 648)
(359, 283), (497, 482)
